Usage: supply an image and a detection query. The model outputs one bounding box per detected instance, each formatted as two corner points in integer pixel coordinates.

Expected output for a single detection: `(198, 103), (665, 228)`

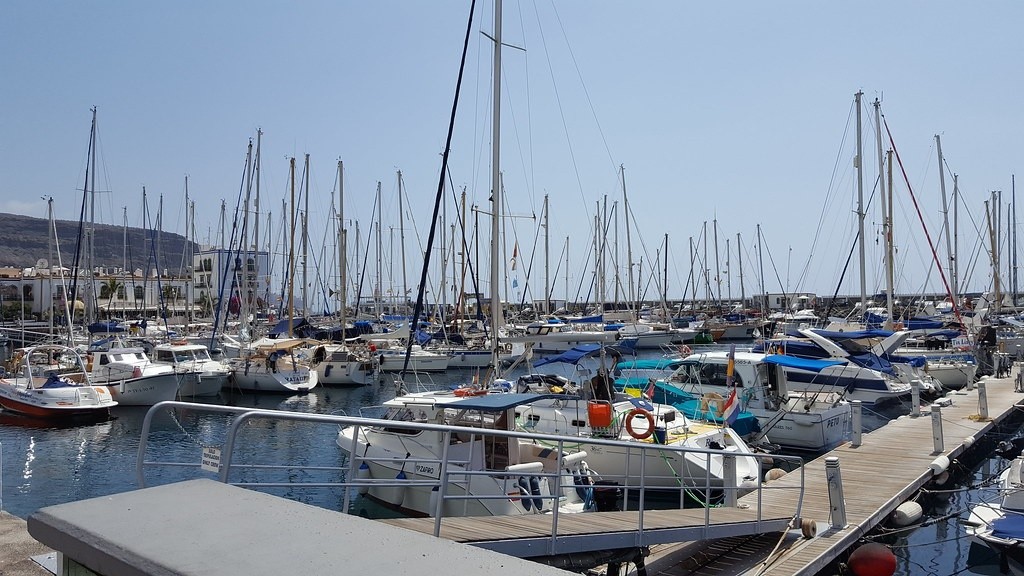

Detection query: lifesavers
(626, 408), (655, 439)
(701, 393), (725, 416)
(679, 344), (692, 358)
(455, 387), (481, 396)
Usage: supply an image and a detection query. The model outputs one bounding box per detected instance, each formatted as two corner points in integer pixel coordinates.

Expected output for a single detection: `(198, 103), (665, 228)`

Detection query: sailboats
(0, 0), (1024, 560)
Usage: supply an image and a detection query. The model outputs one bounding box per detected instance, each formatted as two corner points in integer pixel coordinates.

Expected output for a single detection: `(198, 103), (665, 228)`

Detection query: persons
(895, 316), (904, 331)
(590, 366), (614, 403)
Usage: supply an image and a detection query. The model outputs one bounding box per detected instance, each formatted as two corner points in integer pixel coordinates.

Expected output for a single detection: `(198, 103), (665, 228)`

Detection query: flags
(514, 242), (518, 257)
(723, 386), (740, 426)
